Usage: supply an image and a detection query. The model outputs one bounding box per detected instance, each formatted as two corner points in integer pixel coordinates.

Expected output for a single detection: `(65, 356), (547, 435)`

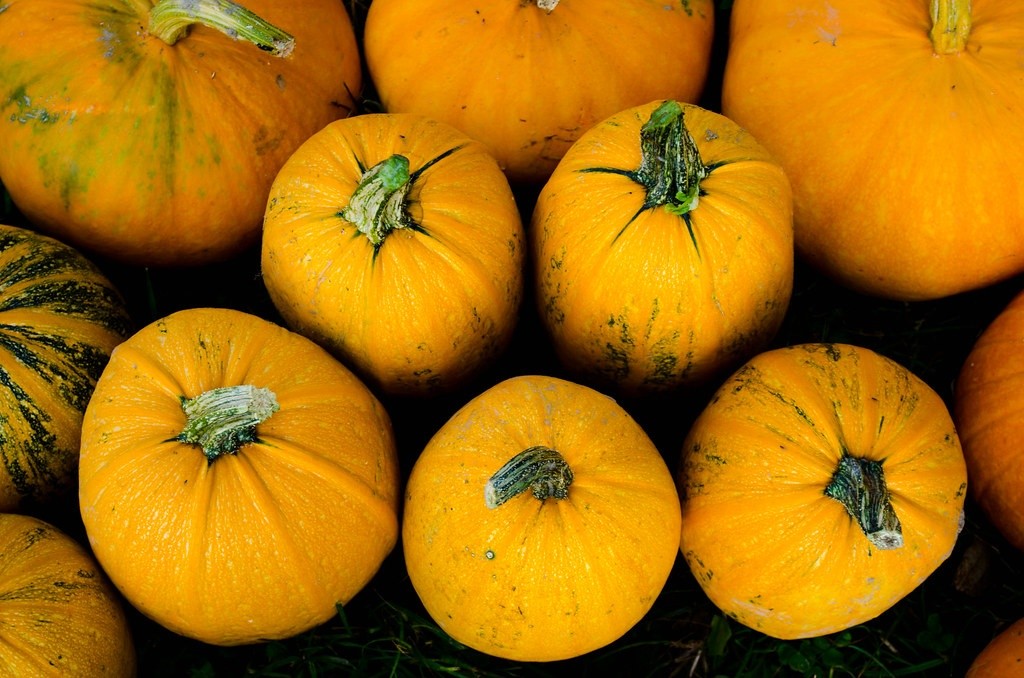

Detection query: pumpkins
(0, 0), (1024, 678)
